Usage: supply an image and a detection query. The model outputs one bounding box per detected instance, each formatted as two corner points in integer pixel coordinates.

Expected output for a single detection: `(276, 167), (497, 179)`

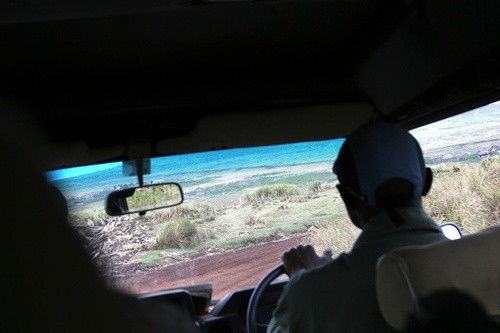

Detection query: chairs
(373, 225), (500, 333)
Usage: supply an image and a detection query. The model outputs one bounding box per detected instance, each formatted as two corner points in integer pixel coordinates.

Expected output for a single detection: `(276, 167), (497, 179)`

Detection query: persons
(0, 149), (153, 333)
(267, 120), (449, 333)
